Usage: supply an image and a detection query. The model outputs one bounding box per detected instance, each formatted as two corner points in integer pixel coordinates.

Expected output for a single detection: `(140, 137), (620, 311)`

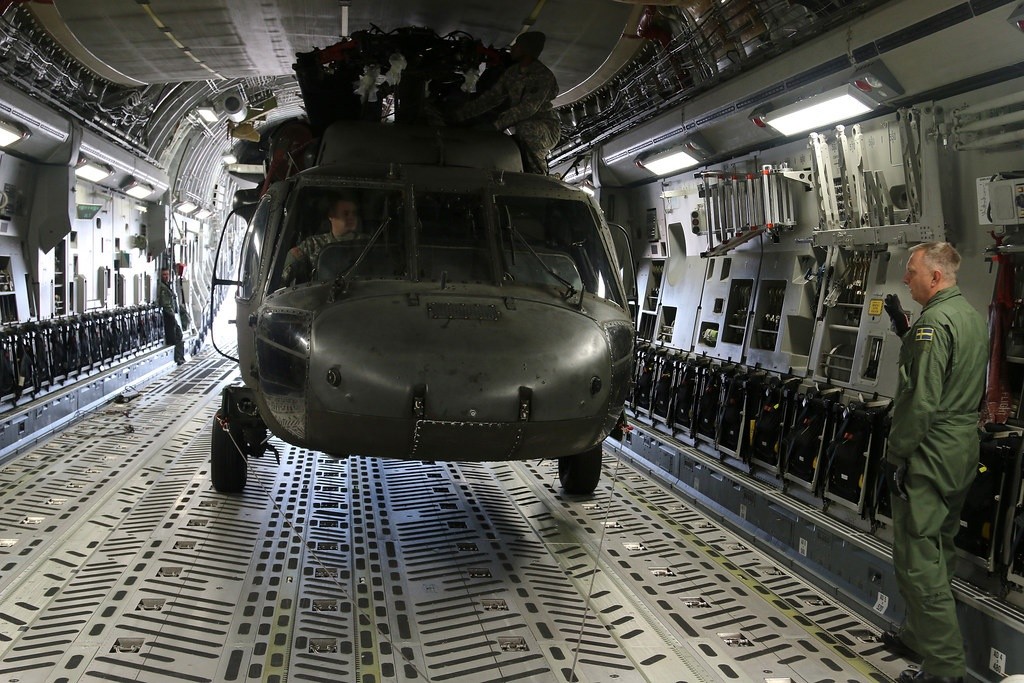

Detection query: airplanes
(210, 24), (641, 500)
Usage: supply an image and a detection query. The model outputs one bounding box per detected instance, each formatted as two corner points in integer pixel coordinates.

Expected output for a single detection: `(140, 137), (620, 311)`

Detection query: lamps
(642, 145), (705, 176)
(766, 84), (881, 137)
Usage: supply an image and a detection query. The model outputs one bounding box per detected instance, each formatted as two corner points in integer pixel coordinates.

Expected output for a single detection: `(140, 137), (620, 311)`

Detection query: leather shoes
(900, 670), (964, 683)
(881, 631), (921, 663)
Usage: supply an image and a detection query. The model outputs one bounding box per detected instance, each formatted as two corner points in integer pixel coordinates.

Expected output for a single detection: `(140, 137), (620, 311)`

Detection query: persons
(160, 267), (185, 365)
(879, 239), (993, 682)
(278, 193), (376, 286)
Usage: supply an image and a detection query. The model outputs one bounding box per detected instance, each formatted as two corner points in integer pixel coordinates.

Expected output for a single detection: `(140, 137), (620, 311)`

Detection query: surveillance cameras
(222, 94), (247, 122)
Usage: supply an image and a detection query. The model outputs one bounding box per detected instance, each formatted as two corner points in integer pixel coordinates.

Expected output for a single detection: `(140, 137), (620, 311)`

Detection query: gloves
(886, 464), (906, 500)
(884, 294), (911, 336)
